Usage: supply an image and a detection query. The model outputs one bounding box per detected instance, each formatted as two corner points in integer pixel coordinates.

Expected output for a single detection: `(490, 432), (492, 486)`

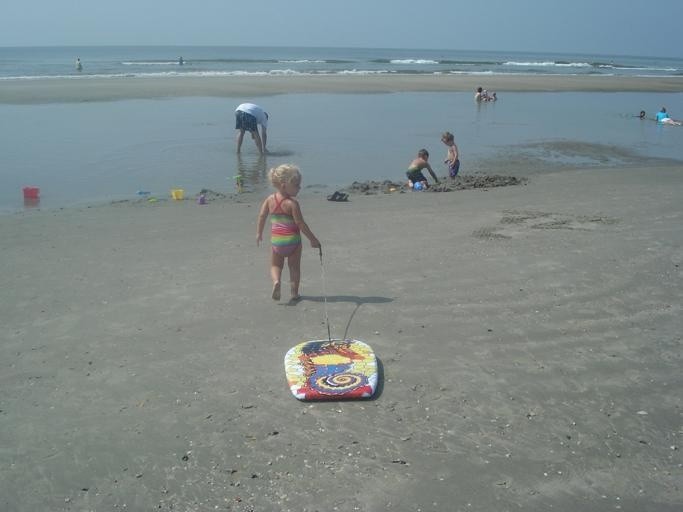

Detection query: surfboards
(285, 339), (378, 401)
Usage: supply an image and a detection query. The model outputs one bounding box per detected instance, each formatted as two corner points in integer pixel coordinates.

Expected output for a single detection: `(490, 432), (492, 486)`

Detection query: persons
(475, 87), (497, 103)
(406, 131), (460, 190)
(639, 111), (646, 120)
(256, 163), (321, 300)
(76, 58), (82, 70)
(654, 107), (681, 126)
(233, 103), (268, 153)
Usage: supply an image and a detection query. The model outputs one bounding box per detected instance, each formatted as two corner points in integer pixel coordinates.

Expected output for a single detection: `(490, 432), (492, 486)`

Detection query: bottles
(413, 181), (424, 191)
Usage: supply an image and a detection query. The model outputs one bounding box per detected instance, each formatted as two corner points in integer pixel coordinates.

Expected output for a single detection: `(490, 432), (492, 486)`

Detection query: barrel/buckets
(171, 190), (183, 200)
